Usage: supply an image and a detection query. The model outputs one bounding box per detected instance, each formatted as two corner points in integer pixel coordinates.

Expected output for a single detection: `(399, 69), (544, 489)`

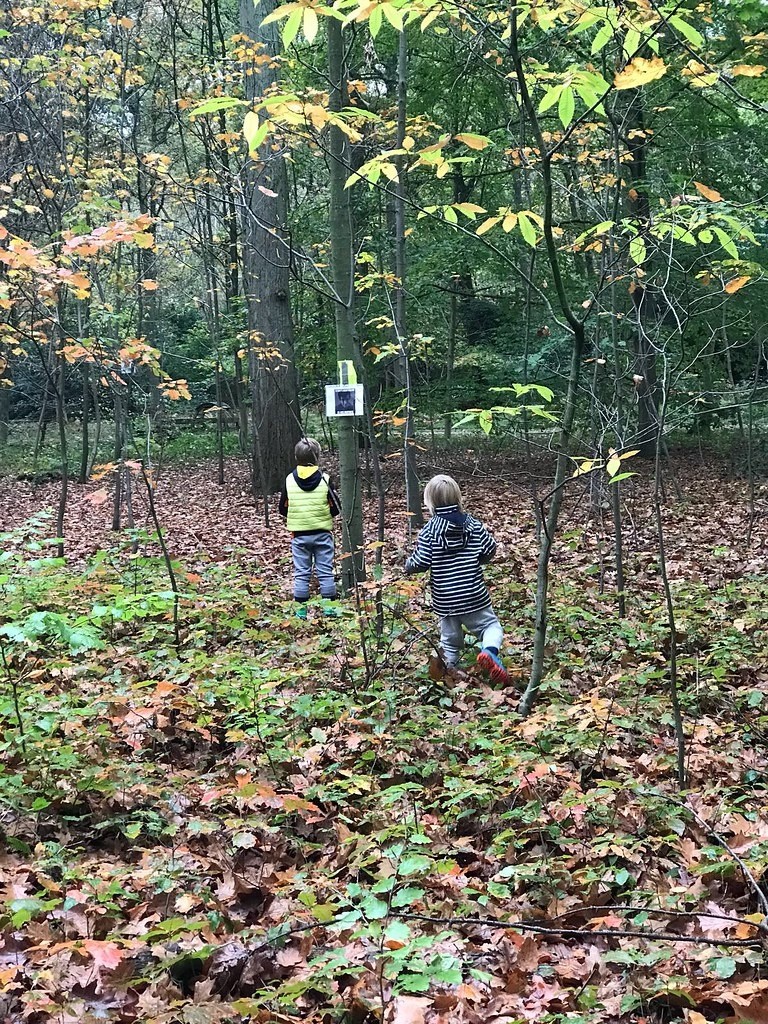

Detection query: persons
(278, 438), (342, 602)
(405, 474), (507, 684)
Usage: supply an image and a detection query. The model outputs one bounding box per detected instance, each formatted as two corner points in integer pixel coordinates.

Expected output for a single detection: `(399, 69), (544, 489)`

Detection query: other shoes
(477, 649), (509, 686)
